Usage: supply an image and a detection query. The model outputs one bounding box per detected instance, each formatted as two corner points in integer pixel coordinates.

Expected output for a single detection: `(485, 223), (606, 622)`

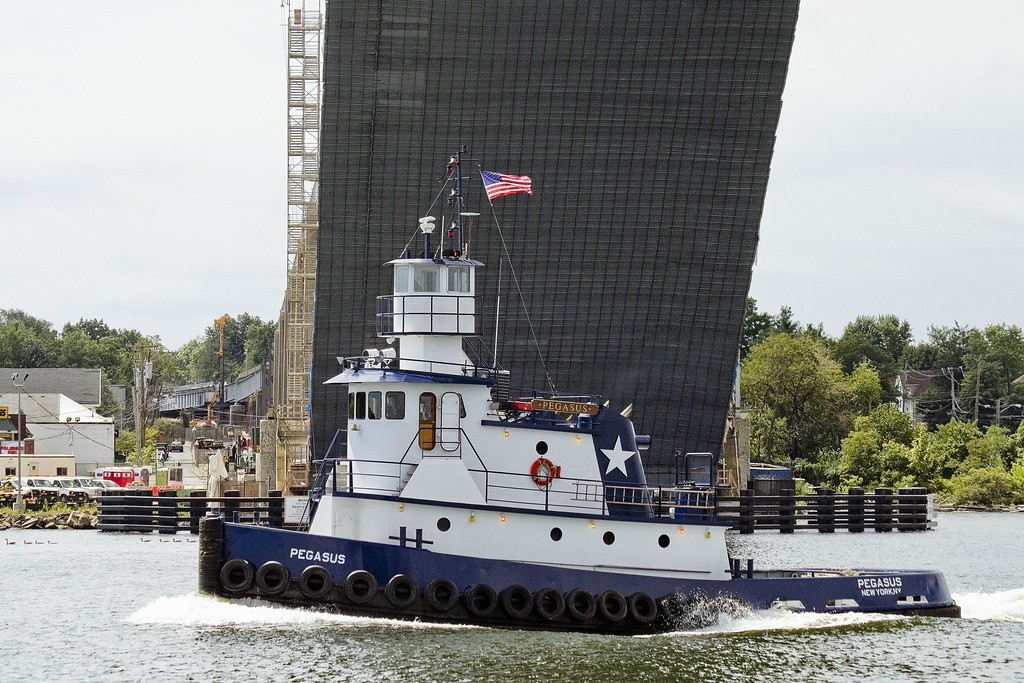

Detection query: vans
(1, 477), (129, 502)
(96, 467), (150, 486)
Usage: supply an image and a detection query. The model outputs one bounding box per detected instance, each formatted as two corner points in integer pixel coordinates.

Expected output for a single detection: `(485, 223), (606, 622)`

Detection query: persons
(349, 394), (375, 420)
(387, 396), (401, 418)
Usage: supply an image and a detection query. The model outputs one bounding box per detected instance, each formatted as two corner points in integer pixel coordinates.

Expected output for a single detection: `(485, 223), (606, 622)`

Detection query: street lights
(12, 372), (30, 502)
(996, 404), (1022, 426)
(975, 403), (990, 424)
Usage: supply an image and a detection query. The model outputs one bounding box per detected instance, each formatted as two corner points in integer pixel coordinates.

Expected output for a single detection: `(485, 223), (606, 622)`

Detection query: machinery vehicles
(237, 431), (256, 474)
(189, 313), (230, 428)
(192, 421), (222, 449)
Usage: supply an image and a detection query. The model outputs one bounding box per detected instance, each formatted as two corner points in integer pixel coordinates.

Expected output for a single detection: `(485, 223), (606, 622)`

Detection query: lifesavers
(423, 577), (460, 613)
(627, 591), (659, 624)
(532, 587), (565, 622)
(341, 569), (378, 605)
(220, 558), (255, 592)
(497, 584), (532, 621)
(298, 565), (334, 600)
(383, 573), (423, 608)
(530, 459), (555, 486)
(564, 587), (595, 621)
(595, 589), (627, 622)
(660, 592), (688, 622)
(463, 584), (498, 618)
(255, 561), (291, 596)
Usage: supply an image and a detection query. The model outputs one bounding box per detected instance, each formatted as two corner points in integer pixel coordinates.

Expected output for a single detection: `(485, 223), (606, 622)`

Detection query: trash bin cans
(750, 462), (795, 523)
(177, 468), (182, 481)
(140, 467), (150, 485)
(170, 468), (176, 481)
(157, 466), (169, 486)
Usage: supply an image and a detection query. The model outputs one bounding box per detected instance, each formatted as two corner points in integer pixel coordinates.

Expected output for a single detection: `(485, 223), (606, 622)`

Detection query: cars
(169, 442), (184, 451)
(152, 447), (168, 469)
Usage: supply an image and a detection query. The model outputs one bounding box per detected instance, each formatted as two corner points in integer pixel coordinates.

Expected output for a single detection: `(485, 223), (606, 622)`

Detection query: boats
(217, 146), (963, 630)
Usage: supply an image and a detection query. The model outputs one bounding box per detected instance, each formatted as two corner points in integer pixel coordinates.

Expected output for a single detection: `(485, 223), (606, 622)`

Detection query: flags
(481, 170), (532, 199)
